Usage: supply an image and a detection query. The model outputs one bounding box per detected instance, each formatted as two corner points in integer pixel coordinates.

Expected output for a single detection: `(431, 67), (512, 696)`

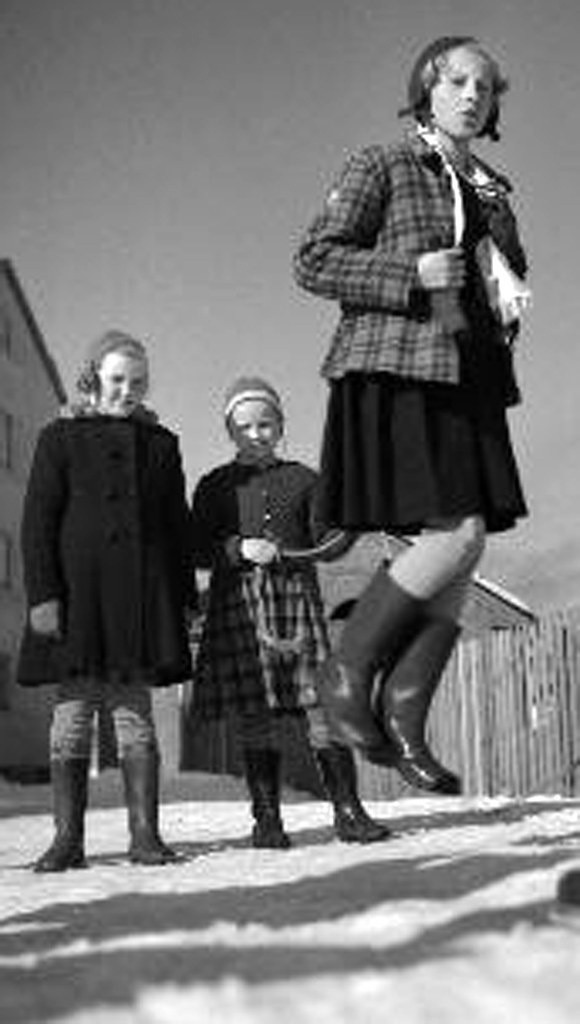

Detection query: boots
(243, 749), (291, 848)
(37, 758), (90, 870)
(311, 747), (389, 842)
(367, 615), (464, 795)
(315, 558), (424, 766)
(120, 757), (176, 865)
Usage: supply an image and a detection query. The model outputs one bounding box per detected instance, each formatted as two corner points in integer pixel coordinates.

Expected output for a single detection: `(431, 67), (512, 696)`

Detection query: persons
(184, 375), (395, 848)
(15, 327), (205, 875)
(292, 34), (530, 797)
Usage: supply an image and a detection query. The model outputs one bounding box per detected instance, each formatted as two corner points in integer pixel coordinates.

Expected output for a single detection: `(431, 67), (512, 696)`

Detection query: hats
(222, 377), (284, 426)
